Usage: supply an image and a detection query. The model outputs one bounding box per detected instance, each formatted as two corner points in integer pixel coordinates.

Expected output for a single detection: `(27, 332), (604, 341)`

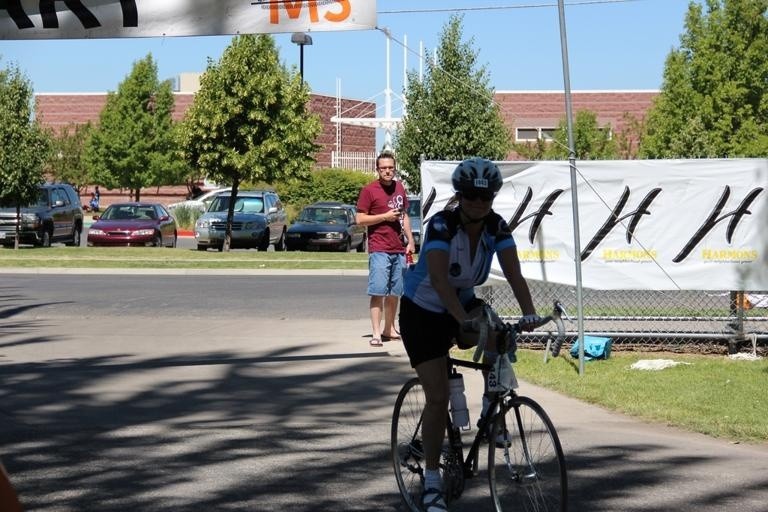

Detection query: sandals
(477, 411), (512, 449)
(420, 487), (449, 511)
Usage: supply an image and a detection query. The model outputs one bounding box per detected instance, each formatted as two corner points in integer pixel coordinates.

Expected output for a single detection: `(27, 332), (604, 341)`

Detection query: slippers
(370, 334), (402, 347)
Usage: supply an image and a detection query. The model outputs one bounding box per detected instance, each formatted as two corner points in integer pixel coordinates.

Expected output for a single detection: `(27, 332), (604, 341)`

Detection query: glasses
(462, 190), (495, 202)
(378, 167), (395, 172)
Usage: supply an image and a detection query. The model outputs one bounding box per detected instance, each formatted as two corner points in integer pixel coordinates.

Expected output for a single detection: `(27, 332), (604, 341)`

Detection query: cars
(86, 202), (178, 249)
(402, 195), (422, 252)
(284, 201), (367, 254)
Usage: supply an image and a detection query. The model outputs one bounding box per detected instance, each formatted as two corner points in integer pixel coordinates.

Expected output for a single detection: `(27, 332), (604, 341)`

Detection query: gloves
(519, 314), (541, 333)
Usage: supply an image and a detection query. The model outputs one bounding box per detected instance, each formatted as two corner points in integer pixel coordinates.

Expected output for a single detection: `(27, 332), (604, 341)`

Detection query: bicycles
(387, 299), (573, 511)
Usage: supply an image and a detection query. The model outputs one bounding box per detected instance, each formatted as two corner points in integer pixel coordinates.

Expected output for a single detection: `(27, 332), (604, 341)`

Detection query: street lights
(291, 32), (314, 85)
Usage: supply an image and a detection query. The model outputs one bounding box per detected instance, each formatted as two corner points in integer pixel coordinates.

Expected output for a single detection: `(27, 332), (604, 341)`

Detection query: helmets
(452, 158), (503, 191)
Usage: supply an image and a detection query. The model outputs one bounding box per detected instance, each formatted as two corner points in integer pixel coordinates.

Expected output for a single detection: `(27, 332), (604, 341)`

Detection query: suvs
(192, 189), (288, 252)
(0, 183), (83, 249)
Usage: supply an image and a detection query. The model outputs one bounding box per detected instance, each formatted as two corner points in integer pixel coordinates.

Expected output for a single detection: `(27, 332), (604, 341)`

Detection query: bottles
(447, 367), (469, 428)
(407, 251), (414, 270)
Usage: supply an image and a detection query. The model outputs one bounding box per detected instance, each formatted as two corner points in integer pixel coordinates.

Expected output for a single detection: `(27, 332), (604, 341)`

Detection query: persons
(394, 157), (546, 512)
(353, 151), (417, 348)
